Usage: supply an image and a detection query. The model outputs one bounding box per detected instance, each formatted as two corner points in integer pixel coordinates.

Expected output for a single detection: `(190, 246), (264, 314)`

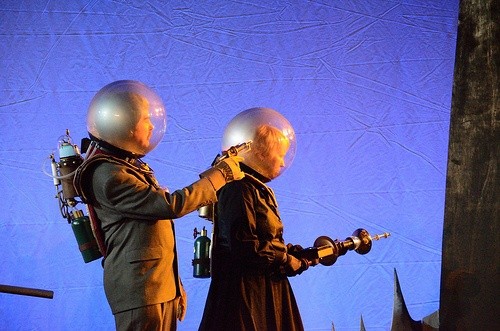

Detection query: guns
(212, 139), (254, 167)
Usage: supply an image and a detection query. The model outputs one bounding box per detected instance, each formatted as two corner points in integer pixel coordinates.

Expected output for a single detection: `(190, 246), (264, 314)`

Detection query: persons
(80, 80), (245, 331)
(198, 107), (304, 331)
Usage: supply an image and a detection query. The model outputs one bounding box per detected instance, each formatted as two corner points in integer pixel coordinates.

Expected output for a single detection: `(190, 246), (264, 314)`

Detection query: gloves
(198, 156), (245, 192)
(285, 254), (302, 278)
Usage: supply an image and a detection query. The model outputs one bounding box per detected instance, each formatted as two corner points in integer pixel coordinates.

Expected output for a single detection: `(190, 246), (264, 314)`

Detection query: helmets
(221, 107), (297, 183)
(86, 79), (168, 158)
